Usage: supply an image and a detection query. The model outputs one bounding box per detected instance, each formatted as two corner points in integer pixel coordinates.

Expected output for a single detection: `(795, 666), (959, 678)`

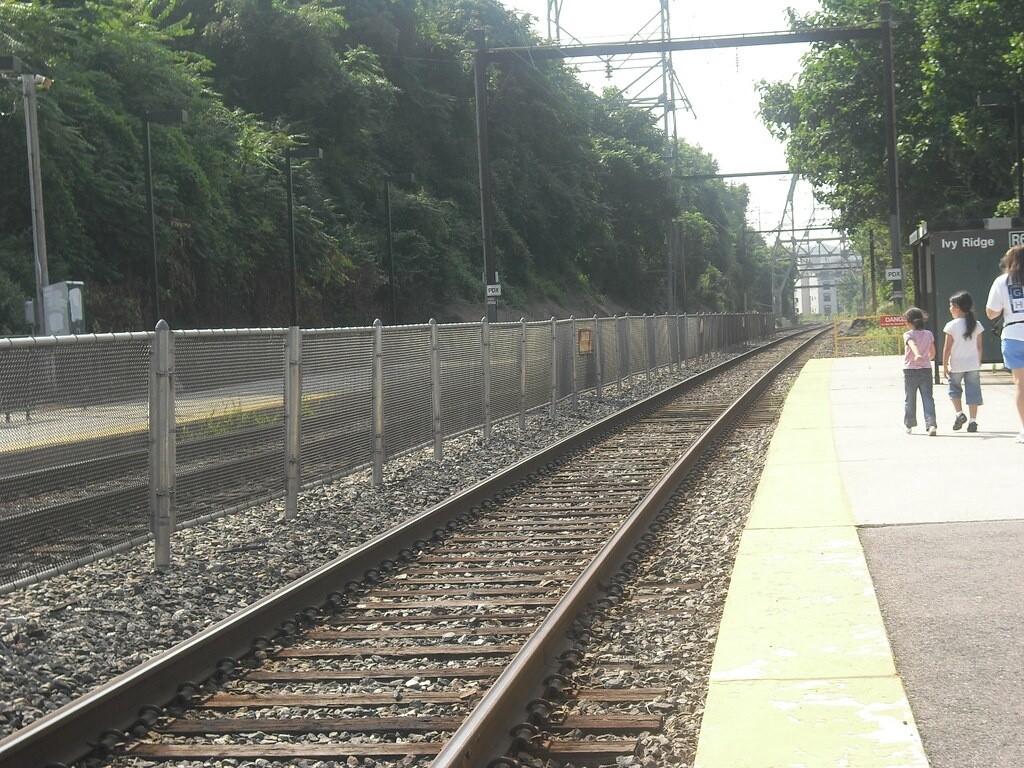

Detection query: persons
(903, 307), (936, 436)
(942, 290), (985, 432)
(985, 244), (1024, 444)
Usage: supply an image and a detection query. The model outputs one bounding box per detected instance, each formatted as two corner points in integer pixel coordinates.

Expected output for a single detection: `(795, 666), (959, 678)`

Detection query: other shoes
(953, 414), (966, 430)
(967, 422), (977, 432)
(903, 425), (912, 434)
(928, 426), (936, 435)
(1016, 432), (1024, 444)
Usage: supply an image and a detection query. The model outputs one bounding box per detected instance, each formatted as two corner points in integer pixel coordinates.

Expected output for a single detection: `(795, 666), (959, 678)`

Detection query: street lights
(284, 146), (325, 325)
(140, 109), (190, 329)
(385, 171), (415, 326)
